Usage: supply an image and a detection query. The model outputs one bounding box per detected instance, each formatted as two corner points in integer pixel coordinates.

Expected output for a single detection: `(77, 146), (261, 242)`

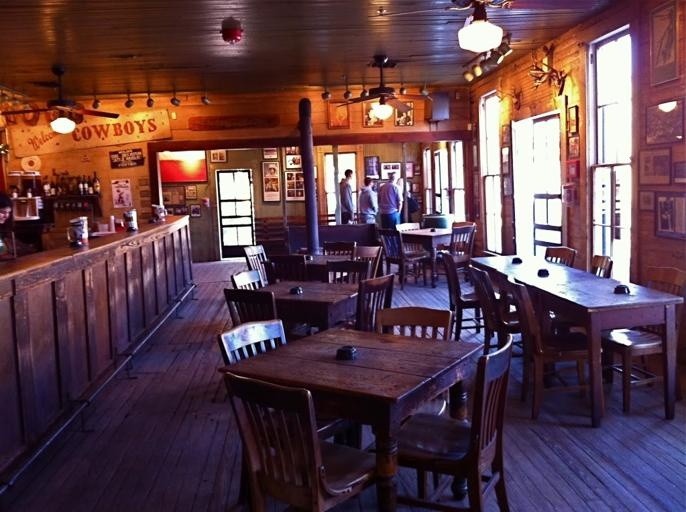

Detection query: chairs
(224, 241), (395, 341)
(600, 265), (686, 412)
(376, 214), (476, 289)
(440, 246), (614, 369)
(375, 307), (452, 340)
(507, 274), (603, 419)
(217, 319), (287, 366)
(223, 371), (376, 511)
(367, 333), (513, 511)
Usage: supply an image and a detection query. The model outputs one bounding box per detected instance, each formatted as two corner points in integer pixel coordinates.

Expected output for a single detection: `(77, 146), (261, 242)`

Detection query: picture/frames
(363, 154), (424, 212)
(471, 141), (481, 219)
(649, 1), (681, 88)
(654, 190), (686, 241)
(392, 100), (415, 128)
(361, 98), (385, 129)
(259, 146), (318, 205)
(638, 189), (657, 213)
(672, 160), (686, 185)
(644, 96), (686, 146)
(637, 147), (673, 187)
(500, 123), (514, 200)
(565, 104), (581, 181)
(326, 100), (351, 131)
(157, 148), (227, 218)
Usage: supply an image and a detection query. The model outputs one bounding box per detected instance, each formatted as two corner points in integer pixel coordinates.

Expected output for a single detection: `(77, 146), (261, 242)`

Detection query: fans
(328, 53), (433, 113)
(367, 0), (523, 23)
(0, 64), (121, 123)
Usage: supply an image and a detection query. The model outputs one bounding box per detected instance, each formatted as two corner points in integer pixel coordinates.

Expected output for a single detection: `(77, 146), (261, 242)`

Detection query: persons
(0, 189), (13, 241)
(360, 171), (421, 257)
(340, 169), (355, 225)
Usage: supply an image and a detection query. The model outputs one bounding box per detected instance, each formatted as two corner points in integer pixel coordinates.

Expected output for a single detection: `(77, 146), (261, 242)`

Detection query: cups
(98, 224), (109, 232)
(66, 215), (90, 247)
(53, 199), (90, 208)
(149, 203), (168, 224)
(120, 208), (139, 231)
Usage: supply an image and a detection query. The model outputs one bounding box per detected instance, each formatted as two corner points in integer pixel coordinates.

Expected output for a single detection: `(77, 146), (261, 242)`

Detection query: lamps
(48, 111), (77, 134)
(463, 32), (515, 82)
(315, 68), (431, 100)
(370, 95), (394, 121)
(454, 10), (505, 55)
(85, 79), (214, 109)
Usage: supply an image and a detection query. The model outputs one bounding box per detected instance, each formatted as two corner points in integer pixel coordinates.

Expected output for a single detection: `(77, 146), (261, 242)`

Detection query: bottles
(26, 187), (33, 197)
(10, 186), (18, 197)
(40, 170), (101, 194)
(108, 215), (116, 232)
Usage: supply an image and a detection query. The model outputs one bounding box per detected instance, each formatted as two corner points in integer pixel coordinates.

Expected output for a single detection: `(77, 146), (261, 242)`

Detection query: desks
(218, 327), (485, 511)
(470, 254), (685, 428)
